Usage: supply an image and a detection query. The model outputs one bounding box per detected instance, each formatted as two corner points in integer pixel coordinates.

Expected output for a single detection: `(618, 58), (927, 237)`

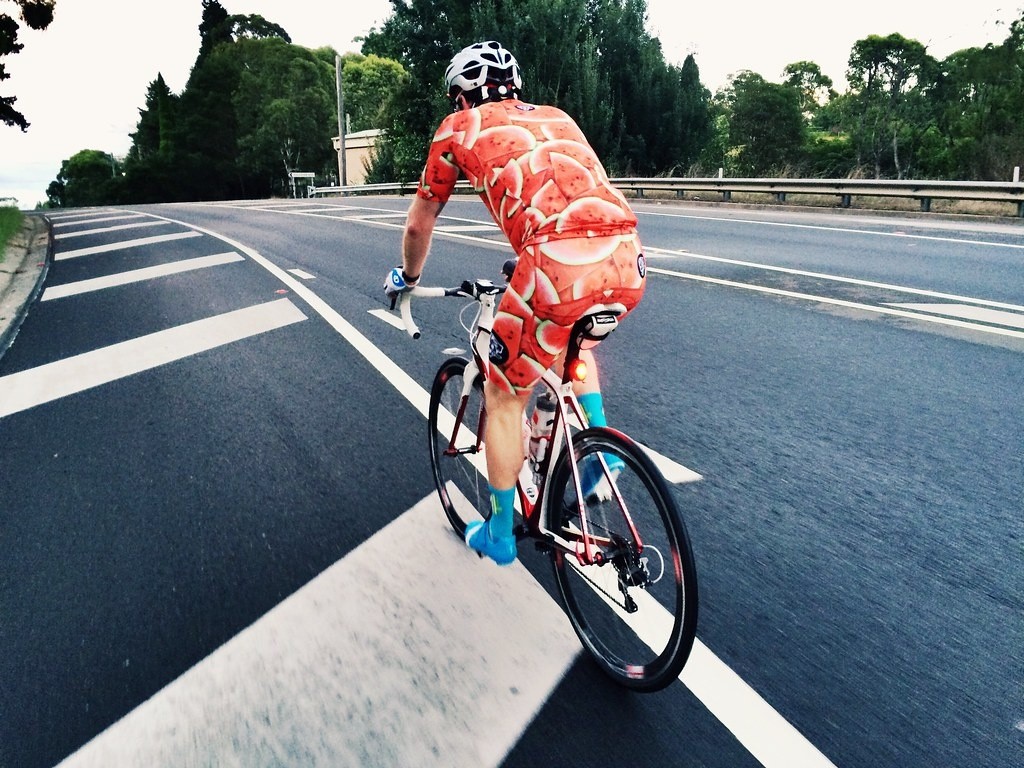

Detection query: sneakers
(465, 519), (518, 566)
(580, 451), (626, 505)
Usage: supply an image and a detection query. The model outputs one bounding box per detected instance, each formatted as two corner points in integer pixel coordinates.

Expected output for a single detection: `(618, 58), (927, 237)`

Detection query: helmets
(444, 40), (523, 101)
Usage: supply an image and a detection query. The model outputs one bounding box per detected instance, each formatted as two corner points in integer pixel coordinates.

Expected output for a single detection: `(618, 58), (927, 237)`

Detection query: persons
(383, 41), (647, 566)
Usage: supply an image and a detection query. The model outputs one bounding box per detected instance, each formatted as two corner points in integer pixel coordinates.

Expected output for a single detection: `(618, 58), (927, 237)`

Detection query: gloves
(383, 267), (421, 298)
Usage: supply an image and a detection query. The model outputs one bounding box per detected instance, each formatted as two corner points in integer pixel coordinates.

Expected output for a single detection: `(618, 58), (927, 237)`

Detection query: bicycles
(390, 253), (701, 694)
